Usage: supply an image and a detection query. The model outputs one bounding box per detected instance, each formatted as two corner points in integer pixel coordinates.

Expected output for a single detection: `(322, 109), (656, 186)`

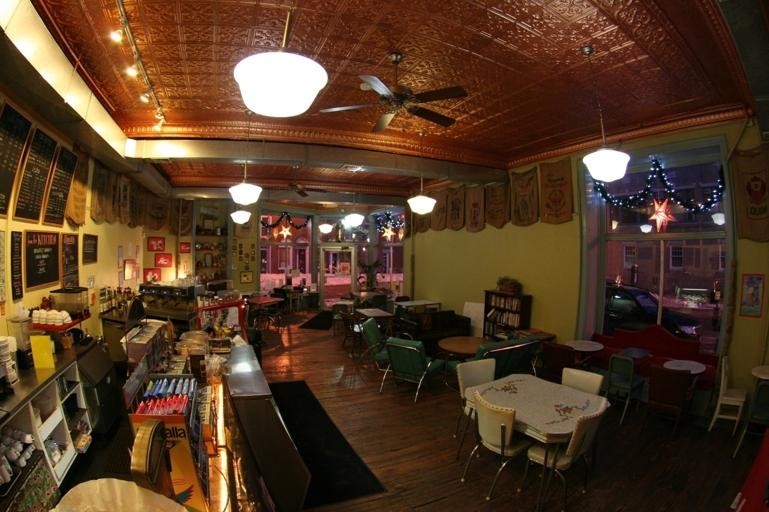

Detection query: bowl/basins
(32, 308), (73, 326)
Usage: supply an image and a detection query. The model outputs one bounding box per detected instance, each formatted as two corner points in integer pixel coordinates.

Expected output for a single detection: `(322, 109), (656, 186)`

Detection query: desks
(248, 297), (284, 329)
(463, 373), (609, 510)
(560, 339), (604, 368)
(438, 336), (500, 380)
(664, 359), (706, 395)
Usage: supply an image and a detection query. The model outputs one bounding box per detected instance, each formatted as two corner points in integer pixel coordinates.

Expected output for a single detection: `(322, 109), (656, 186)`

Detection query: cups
(0, 425), (37, 486)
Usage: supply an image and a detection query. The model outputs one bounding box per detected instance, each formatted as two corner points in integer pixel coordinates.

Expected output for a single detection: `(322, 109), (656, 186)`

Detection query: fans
(275, 166), (329, 205)
(319, 53), (469, 137)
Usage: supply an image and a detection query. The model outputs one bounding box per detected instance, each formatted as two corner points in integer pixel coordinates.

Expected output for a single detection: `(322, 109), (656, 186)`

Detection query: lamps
(611, 217), (622, 235)
(318, 223), (333, 234)
(228, 111), (263, 206)
(233, 1), (329, 119)
(344, 193), (365, 228)
(580, 46), (631, 183)
(406, 131), (438, 216)
(229, 205), (251, 225)
(636, 222), (656, 238)
(709, 209), (728, 229)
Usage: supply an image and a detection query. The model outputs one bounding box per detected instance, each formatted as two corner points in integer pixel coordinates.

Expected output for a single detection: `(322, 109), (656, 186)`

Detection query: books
(485, 295), (520, 339)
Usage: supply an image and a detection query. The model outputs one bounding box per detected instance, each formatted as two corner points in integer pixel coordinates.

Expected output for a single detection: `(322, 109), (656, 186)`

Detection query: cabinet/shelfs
(1, 358), (93, 511)
(483, 290), (533, 336)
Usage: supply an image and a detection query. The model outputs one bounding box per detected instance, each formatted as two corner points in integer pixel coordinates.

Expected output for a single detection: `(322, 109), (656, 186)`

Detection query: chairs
(289, 290), (300, 311)
(445, 336), (540, 382)
(646, 363), (691, 436)
(560, 367), (605, 394)
(326, 292), (473, 364)
(607, 355), (646, 423)
(708, 354), (746, 435)
(379, 336), (446, 403)
(461, 390), (532, 500)
(521, 408), (608, 510)
(451, 359), (503, 460)
(550, 342), (593, 383)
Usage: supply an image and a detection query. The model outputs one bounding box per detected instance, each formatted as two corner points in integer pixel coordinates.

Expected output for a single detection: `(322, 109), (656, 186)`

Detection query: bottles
(41, 295), (55, 309)
(0, 337), (19, 388)
(21, 305), (39, 319)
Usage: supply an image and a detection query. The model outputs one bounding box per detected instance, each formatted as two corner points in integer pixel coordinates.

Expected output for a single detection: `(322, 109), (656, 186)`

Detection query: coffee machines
(136, 283), (196, 319)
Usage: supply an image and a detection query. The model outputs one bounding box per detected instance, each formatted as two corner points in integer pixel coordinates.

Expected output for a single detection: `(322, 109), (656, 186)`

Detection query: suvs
(605, 283), (703, 344)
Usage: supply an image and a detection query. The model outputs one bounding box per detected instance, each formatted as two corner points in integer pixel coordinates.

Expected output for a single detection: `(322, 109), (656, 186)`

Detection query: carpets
(298, 308), (339, 332)
(269, 364), (387, 510)
